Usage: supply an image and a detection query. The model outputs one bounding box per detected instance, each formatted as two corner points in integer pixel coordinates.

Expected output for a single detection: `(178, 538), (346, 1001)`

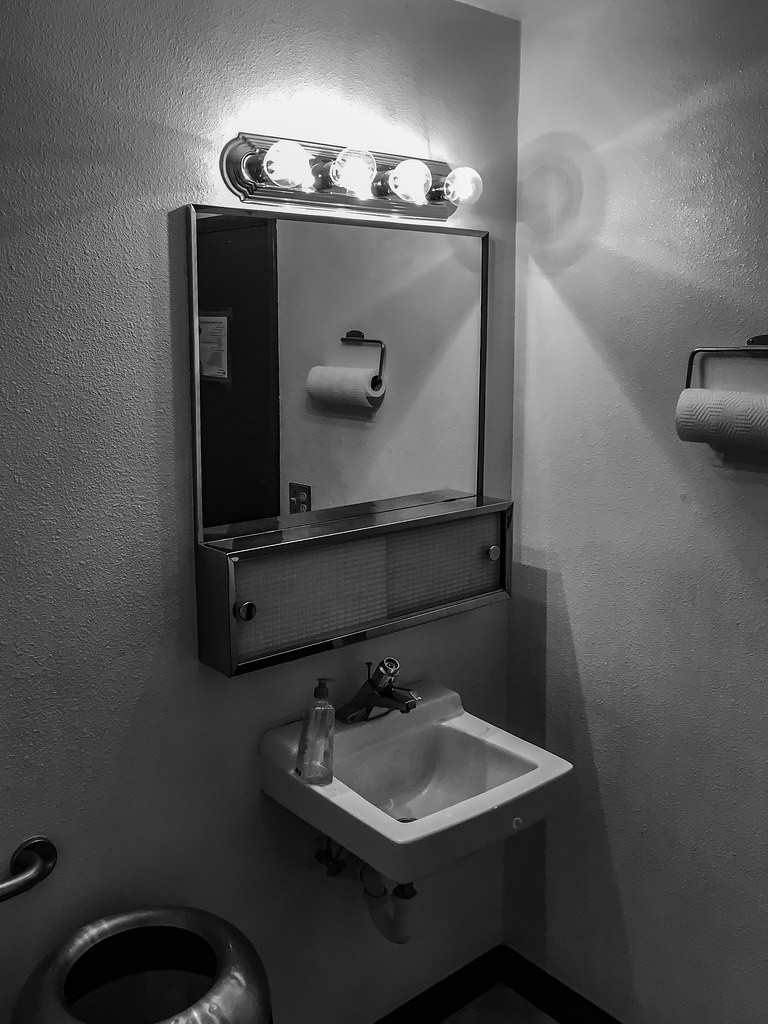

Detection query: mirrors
(184, 203), (489, 552)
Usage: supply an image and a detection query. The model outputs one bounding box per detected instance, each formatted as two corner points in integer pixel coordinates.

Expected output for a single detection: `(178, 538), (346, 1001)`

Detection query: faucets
(334, 657), (417, 723)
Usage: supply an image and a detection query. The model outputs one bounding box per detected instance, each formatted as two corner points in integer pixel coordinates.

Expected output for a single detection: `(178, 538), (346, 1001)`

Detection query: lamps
(220, 133), (483, 222)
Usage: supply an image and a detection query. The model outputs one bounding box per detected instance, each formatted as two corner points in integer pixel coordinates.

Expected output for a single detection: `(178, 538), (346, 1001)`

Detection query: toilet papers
(674, 386), (768, 451)
(305, 365), (387, 407)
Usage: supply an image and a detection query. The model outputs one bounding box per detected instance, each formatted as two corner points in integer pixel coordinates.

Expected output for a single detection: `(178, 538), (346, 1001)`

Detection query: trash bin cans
(23, 904), (276, 1024)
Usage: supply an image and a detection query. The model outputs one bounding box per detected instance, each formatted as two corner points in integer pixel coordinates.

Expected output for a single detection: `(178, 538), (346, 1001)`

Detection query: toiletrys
(293, 677), (338, 787)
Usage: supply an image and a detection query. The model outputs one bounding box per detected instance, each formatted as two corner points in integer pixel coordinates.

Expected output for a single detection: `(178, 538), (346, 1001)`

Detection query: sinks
(257, 678), (572, 884)
